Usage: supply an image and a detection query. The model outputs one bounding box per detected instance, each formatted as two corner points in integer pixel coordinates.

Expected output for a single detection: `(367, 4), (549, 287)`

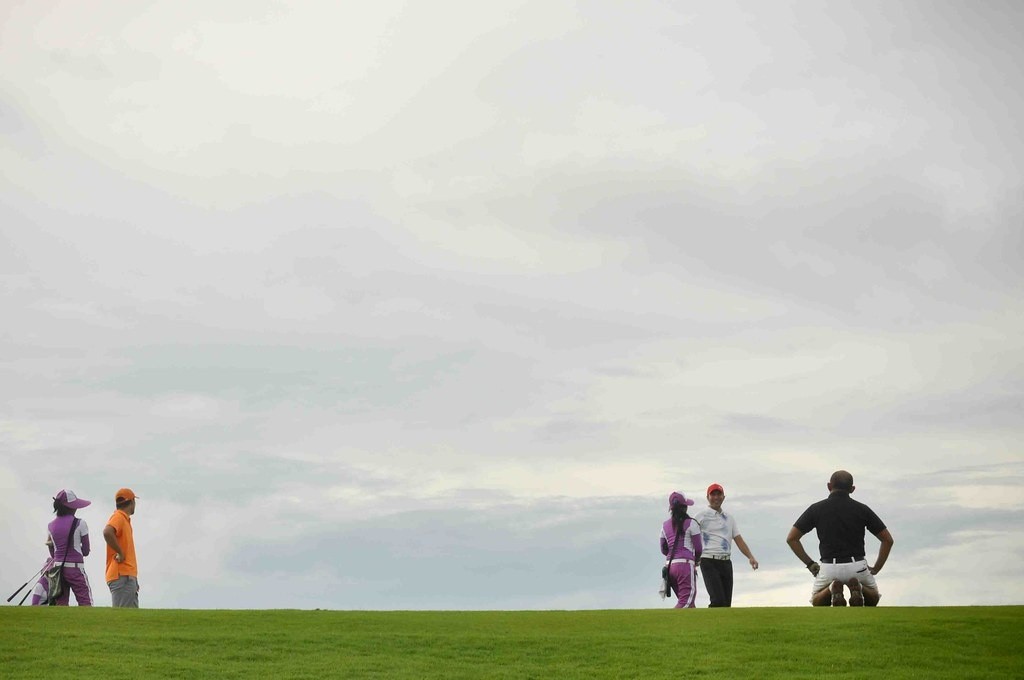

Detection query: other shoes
(848, 578), (864, 607)
(829, 579), (847, 607)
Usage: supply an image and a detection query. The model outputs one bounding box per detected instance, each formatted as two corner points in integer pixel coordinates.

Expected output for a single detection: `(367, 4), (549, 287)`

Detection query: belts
(665, 559), (695, 565)
(701, 554), (730, 561)
(822, 555), (863, 563)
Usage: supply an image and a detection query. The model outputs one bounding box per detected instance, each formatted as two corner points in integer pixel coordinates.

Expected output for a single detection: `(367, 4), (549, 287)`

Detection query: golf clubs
(6, 558), (54, 606)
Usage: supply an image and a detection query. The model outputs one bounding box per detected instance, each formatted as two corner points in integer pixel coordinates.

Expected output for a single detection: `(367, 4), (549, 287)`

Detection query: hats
(668, 493), (694, 513)
(115, 488), (139, 504)
(707, 483), (724, 495)
(41, 557), (54, 571)
(55, 489), (91, 510)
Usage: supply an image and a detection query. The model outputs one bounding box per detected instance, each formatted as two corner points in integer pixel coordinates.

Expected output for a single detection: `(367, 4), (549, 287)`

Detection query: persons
(693, 484), (759, 608)
(102, 488), (141, 608)
(32, 489), (91, 607)
(659, 490), (704, 608)
(786, 470), (894, 607)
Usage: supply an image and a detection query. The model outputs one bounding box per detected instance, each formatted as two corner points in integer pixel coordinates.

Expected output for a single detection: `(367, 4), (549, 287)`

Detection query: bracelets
(807, 561), (814, 568)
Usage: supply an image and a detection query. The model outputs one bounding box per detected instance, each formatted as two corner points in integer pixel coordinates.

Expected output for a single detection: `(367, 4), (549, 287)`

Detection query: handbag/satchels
(47, 566), (64, 599)
(662, 567), (671, 597)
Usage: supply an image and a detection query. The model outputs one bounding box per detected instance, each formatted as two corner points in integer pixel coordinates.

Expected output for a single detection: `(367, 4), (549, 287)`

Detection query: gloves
(807, 561), (820, 577)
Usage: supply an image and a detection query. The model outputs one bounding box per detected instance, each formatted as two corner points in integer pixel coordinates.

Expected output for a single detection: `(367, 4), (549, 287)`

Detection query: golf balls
(115, 553), (119, 559)
